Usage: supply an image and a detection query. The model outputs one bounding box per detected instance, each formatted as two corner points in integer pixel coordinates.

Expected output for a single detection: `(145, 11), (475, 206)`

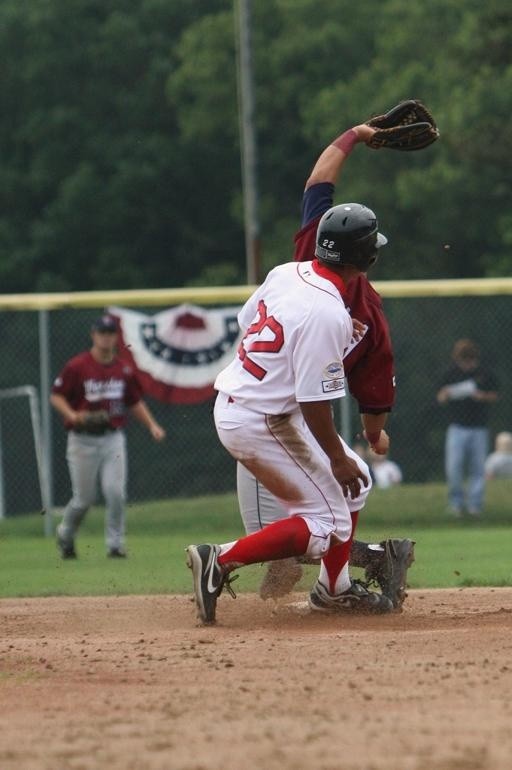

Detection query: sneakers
(107, 546), (126, 560)
(185, 537), (419, 624)
(56, 527), (76, 562)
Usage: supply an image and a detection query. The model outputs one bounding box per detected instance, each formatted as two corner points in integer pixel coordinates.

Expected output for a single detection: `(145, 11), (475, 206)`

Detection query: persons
(434, 338), (500, 524)
(485, 428), (512, 480)
(186, 202), (391, 624)
(367, 446), (403, 488)
(354, 445), (376, 488)
(49, 314), (167, 560)
(236, 99), (439, 614)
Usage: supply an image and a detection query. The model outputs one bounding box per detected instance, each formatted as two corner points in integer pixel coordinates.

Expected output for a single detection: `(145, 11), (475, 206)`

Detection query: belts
(75, 426), (116, 436)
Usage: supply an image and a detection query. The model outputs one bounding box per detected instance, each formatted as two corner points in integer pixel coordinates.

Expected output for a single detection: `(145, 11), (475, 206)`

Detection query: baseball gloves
(76, 410), (110, 436)
(366, 99), (439, 151)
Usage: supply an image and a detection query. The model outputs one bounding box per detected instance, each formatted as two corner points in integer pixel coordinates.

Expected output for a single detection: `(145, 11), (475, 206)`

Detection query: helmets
(311, 201), (387, 271)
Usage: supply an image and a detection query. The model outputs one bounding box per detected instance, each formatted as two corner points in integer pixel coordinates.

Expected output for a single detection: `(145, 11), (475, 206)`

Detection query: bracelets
(332, 128), (358, 154)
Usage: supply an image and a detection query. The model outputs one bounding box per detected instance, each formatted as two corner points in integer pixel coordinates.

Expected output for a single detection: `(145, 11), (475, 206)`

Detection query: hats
(91, 316), (116, 333)
